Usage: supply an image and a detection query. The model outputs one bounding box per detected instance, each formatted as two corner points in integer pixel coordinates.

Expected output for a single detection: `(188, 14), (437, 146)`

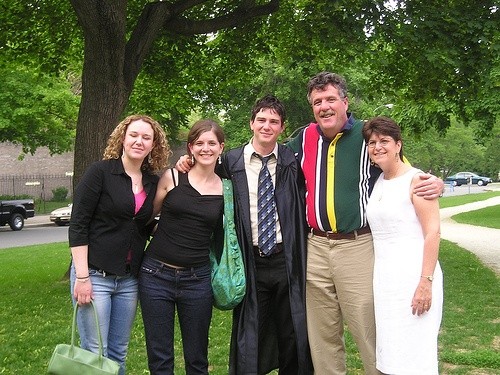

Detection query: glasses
(365, 137), (393, 148)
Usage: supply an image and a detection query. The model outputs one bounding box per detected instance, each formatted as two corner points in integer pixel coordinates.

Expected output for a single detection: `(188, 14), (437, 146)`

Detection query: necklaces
(132, 178), (141, 186)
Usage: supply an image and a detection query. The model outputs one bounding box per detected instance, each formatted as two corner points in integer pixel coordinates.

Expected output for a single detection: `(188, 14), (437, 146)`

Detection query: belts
(88, 262), (131, 278)
(253, 243), (284, 257)
(308, 225), (371, 240)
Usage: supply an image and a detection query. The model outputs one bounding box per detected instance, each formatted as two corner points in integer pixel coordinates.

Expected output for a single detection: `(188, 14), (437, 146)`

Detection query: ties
(252, 152), (277, 254)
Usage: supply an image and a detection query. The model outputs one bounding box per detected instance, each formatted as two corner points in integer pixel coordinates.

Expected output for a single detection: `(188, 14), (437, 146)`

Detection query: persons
(68, 115), (173, 375)
(285, 71), (446, 375)
(137, 119), (226, 375)
(362, 115), (443, 375)
(175, 95), (314, 375)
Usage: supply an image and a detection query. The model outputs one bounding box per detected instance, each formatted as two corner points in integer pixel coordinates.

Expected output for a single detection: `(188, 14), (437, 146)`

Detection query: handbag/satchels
(45, 297), (122, 375)
(208, 177), (247, 311)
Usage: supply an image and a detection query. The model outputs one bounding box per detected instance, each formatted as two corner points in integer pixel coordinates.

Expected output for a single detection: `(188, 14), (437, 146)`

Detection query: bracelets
(439, 187), (445, 197)
(76, 275), (90, 283)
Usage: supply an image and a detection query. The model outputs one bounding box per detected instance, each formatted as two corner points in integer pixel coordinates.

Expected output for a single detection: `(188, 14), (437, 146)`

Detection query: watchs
(421, 275), (433, 281)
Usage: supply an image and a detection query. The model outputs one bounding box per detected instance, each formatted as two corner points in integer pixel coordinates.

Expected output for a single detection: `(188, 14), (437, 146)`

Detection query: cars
(50, 203), (73, 226)
(445, 172), (492, 186)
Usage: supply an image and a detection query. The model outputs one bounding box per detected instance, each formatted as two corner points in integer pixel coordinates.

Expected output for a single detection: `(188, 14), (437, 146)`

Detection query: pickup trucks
(0, 199), (35, 230)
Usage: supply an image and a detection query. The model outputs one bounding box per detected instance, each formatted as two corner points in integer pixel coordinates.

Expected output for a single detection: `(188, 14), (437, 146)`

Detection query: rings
(424, 304), (428, 307)
